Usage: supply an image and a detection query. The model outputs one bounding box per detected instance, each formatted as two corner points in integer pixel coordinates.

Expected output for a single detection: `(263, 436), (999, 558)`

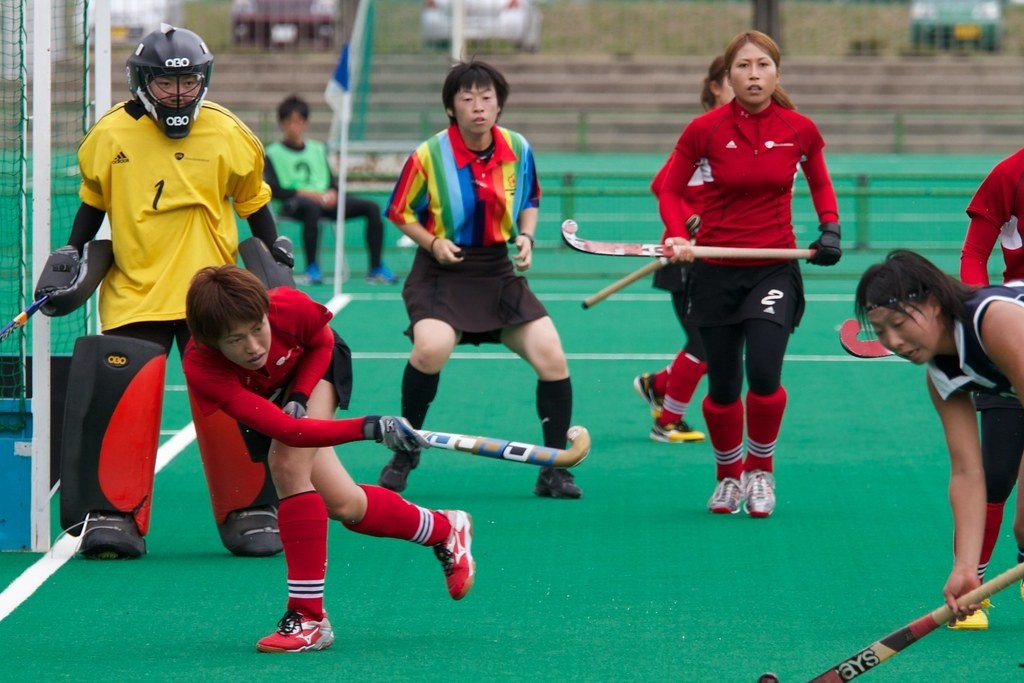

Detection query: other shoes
(946, 610), (988, 630)
(1021, 582), (1024, 597)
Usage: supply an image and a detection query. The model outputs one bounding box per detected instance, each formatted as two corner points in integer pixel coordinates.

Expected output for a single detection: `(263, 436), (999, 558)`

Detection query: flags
(322, 0), (373, 176)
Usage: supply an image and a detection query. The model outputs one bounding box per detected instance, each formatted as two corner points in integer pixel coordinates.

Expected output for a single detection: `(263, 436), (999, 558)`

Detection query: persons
(854, 248), (1024, 627)
(264, 97), (400, 284)
(379, 56), (584, 499)
(947, 149), (1024, 628)
(35, 22), (296, 557)
(657, 30), (843, 517)
(634, 55), (736, 445)
(182, 263), (475, 650)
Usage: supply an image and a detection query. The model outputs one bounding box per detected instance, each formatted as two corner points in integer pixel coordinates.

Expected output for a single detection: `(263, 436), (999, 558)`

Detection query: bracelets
(520, 232), (534, 248)
(431, 237), (438, 254)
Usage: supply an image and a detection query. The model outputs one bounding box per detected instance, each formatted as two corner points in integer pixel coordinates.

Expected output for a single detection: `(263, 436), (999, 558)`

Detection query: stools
(271, 209), (339, 284)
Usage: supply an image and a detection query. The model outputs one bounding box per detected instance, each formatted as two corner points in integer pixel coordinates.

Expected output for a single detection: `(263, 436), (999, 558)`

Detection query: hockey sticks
(581, 255), (669, 310)
(412, 425), (592, 469)
(0, 293), (52, 342)
(758, 561), (1024, 683)
(560, 217), (817, 259)
(837, 318), (897, 358)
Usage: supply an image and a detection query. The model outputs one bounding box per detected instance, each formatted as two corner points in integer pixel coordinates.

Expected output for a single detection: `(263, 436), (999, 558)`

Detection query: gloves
(375, 414), (431, 453)
(806, 223), (841, 266)
(281, 401), (306, 418)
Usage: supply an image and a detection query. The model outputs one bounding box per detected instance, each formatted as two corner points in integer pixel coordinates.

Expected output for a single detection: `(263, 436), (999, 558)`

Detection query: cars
(420, 0), (540, 52)
(228, 0), (342, 52)
(908, 0), (1004, 55)
(73, 0), (186, 49)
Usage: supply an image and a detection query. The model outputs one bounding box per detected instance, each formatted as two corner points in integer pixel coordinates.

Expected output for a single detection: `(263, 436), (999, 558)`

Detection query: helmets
(125, 21), (214, 138)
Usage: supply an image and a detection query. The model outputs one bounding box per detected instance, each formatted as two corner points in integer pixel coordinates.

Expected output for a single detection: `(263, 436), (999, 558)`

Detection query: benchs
(0, 51), (1023, 161)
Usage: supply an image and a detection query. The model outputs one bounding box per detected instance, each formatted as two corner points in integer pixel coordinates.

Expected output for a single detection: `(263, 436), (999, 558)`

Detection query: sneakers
(534, 467), (581, 498)
(435, 510), (476, 600)
(303, 262), (322, 283)
(634, 373), (664, 418)
(650, 417), (705, 443)
(708, 463), (745, 514)
(257, 609), (334, 653)
(378, 450), (421, 492)
(365, 267), (400, 285)
(743, 469), (775, 516)
